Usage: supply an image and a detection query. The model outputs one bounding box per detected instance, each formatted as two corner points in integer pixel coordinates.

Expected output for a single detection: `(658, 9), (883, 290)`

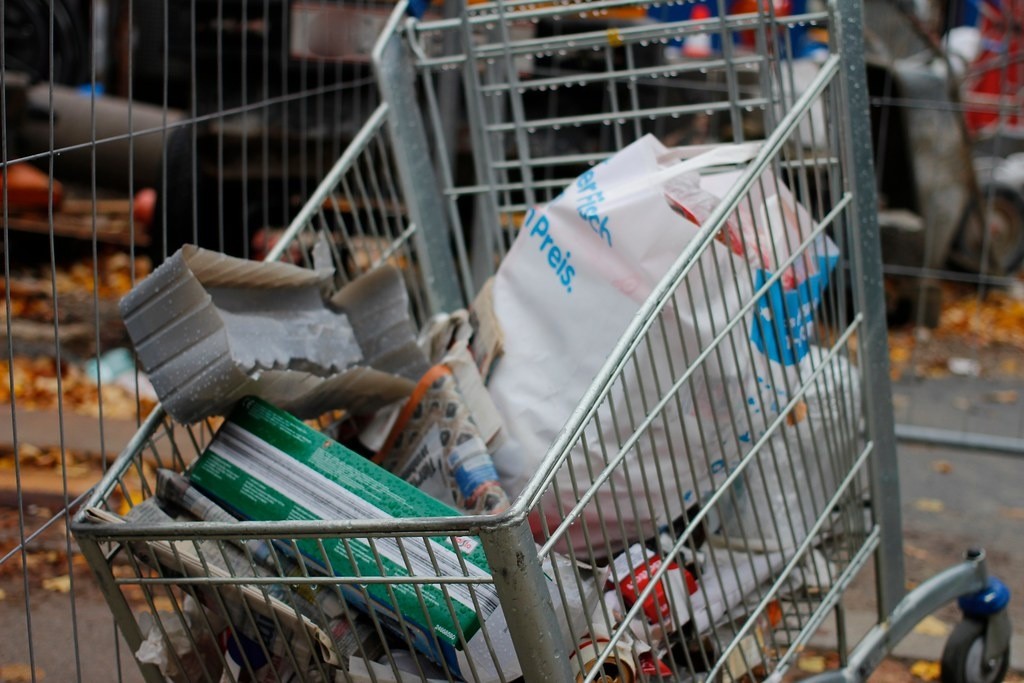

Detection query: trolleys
(68, 0), (1012, 679)
